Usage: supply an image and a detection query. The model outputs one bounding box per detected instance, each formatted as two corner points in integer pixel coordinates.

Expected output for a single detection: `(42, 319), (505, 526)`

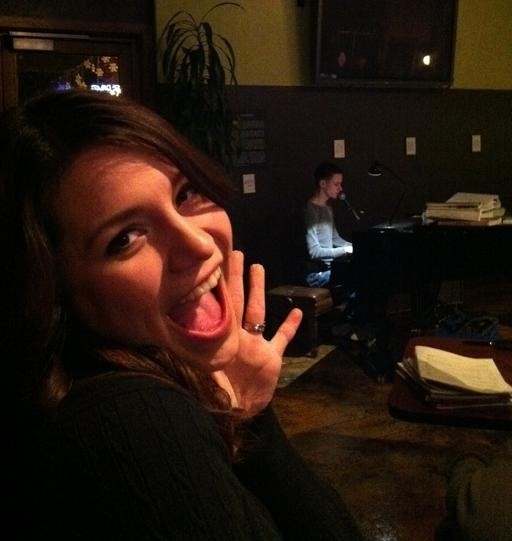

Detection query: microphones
(338, 193), (361, 222)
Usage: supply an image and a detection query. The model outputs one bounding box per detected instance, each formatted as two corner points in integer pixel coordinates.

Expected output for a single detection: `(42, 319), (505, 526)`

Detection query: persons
(303, 162), (363, 321)
(4, 84), (366, 540)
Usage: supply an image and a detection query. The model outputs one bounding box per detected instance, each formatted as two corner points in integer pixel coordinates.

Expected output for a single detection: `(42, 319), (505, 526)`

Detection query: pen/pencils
(462, 339), (492, 346)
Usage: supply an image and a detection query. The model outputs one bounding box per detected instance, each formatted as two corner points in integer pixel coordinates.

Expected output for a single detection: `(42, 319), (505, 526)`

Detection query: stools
(267, 284), (339, 358)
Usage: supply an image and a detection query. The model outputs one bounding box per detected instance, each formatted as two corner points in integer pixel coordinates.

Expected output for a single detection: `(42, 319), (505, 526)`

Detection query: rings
(242, 320), (265, 332)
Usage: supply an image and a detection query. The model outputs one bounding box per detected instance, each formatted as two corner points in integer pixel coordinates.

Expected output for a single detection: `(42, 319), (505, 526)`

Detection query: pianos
(329, 213), (511, 359)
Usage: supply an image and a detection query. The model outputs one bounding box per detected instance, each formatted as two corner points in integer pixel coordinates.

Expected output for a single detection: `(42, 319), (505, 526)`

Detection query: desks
(385, 336), (511, 431)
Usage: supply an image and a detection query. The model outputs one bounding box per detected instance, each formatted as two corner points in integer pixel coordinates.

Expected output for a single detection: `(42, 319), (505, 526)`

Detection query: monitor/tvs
(315, 3), (461, 91)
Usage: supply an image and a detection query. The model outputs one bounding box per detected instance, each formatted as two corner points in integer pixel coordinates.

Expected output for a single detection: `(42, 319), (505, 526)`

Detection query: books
(424, 191), (506, 226)
(395, 344), (512, 410)
